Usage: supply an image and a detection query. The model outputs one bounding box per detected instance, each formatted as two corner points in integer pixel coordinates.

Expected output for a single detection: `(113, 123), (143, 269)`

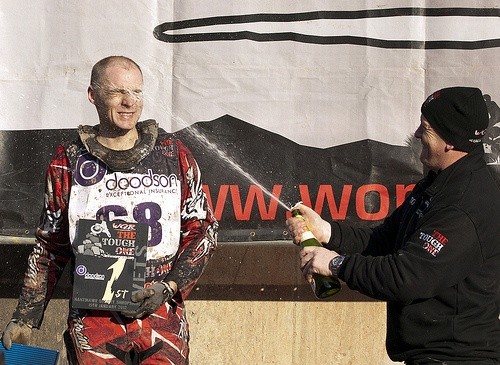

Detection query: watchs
(329, 255), (345, 278)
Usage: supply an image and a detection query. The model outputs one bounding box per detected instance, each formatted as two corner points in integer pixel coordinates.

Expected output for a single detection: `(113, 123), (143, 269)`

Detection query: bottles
(291, 207), (341, 300)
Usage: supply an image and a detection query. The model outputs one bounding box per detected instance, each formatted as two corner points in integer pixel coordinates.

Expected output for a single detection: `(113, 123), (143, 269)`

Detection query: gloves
(121, 281), (172, 319)
(1, 319), (32, 349)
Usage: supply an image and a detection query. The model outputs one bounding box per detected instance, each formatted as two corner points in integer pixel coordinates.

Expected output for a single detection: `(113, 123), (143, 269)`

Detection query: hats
(420, 87), (490, 152)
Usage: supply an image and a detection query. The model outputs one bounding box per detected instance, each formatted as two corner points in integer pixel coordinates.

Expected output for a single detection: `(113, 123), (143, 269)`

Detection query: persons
(286, 87), (500, 365)
(2, 56), (218, 365)
